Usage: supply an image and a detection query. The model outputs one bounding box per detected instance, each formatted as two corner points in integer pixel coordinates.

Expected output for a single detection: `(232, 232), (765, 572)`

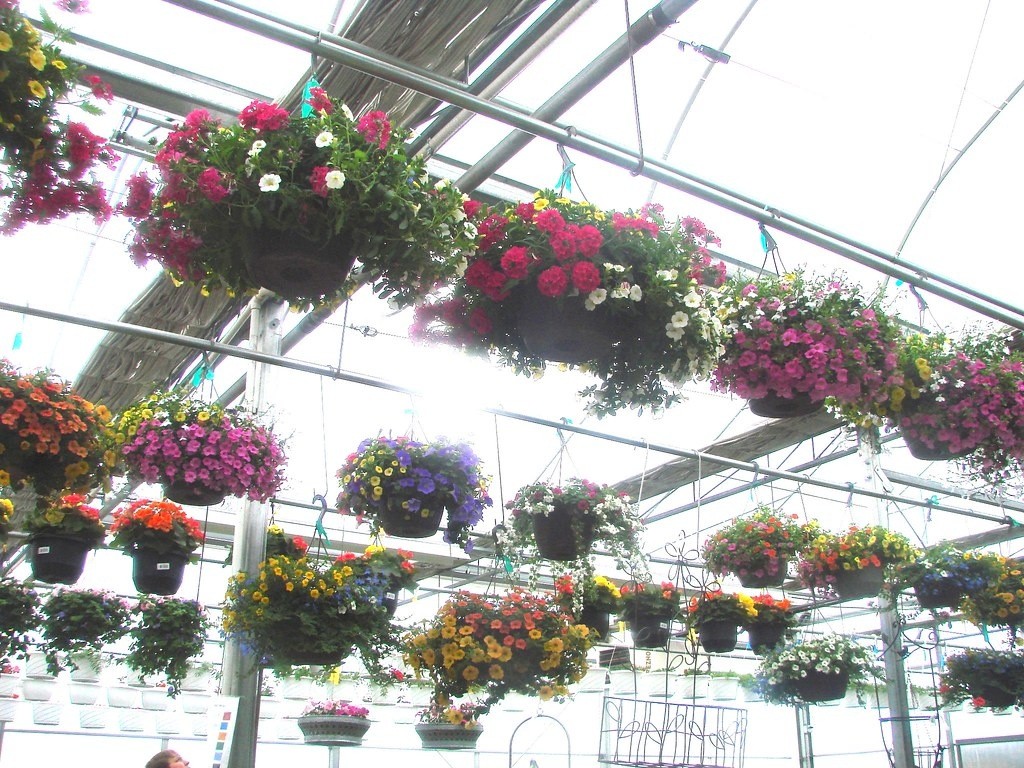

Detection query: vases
(380, 586), (399, 616)
(745, 620), (784, 655)
(898, 424), (977, 460)
(378, 494), (444, 539)
(795, 666), (848, 700)
(268, 622), (343, 665)
(241, 225), (356, 298)
(131, 545), (187, 596)
(161, 477), (227, 506)
(829, 554), (882, 598)
(35, 461), (69, 494)
(570, 608), (609, 640)
(747, 390), (825, 418)
(512, 295), (612, 364)
(413, 723), (483, 749)
(966, 673), (1018, 706)
(732, 554), (788, 588)
(295, 715), (372, 745)
(533, 510), (597, 561)
(982, 610), (1021, 626)
(913, 577), (960, 608)
(31, 534), (91, 586)
(628, 613), (670, 649)
(698, 619), (737, 653)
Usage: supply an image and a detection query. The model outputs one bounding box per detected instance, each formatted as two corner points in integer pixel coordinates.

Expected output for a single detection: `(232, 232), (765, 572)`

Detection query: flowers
(0, 0), (1024, 730)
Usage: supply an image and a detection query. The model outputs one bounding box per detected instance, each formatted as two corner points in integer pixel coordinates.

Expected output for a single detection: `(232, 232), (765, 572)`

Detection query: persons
(146, 749), (191, 768)
